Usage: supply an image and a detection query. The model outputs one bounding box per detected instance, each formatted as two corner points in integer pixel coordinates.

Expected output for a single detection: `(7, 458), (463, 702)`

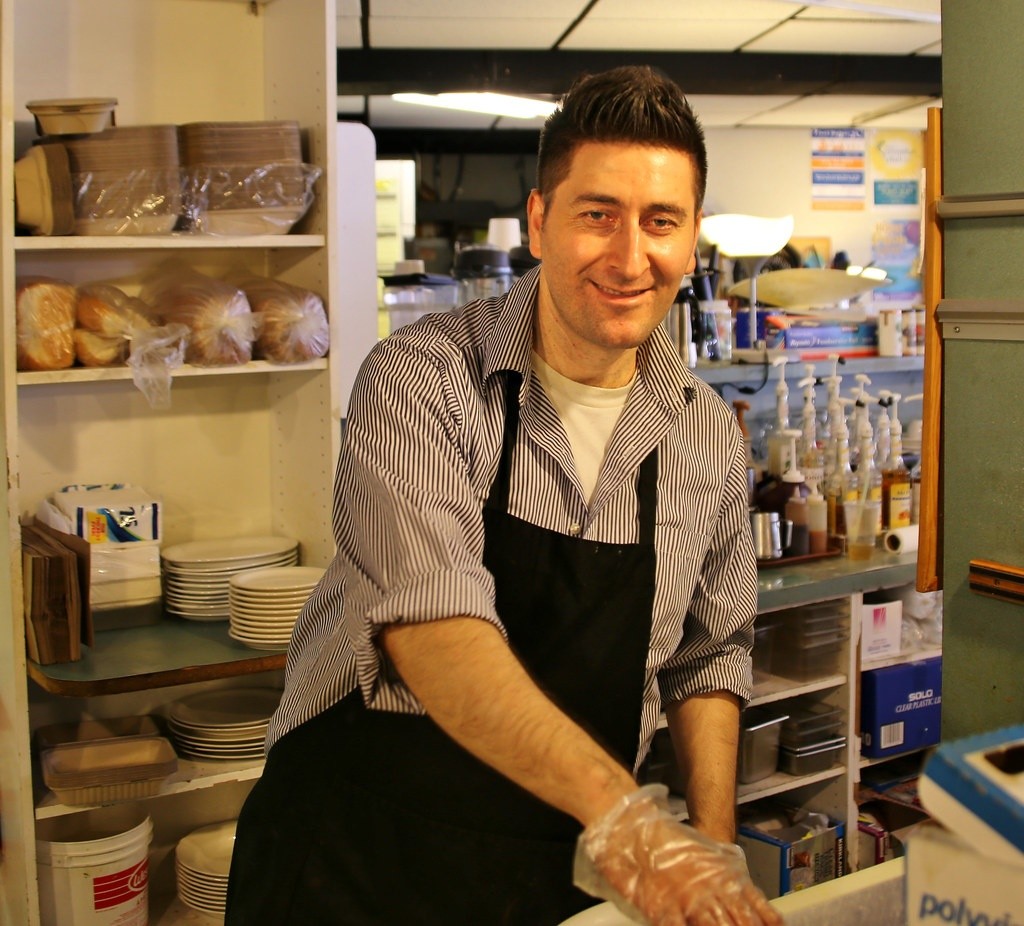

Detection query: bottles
(784, 485), (808, 556)
(806, 485), (828, 554)
(698, 299), (733, 362)
(879, 469), (912, 527)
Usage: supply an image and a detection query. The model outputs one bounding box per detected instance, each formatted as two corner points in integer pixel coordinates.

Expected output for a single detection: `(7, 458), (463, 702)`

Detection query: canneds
(878, 308), (925, 358)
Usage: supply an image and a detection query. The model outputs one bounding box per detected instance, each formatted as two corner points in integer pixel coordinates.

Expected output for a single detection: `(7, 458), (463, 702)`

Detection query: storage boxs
(732, 589), (1024, 925)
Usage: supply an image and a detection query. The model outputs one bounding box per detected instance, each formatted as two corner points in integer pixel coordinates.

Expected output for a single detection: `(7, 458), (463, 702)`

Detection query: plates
(168, 684), (284, 761)
(175, 818), (238, 920)
(160, 535), (328, 651)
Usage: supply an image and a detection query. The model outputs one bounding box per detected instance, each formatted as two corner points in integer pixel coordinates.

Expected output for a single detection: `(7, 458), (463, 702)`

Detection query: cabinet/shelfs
(0, 0), (348, 926)
(623, 576), (942, 903)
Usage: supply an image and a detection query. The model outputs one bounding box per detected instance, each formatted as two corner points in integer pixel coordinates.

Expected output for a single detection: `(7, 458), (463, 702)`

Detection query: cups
(877, 303), (925, 358)
(843, 500), (882, 561)
(750, 510), (793, 560)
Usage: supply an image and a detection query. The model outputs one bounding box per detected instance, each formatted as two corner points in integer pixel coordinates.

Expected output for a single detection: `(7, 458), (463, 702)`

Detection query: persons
(225, 63), (787, 926)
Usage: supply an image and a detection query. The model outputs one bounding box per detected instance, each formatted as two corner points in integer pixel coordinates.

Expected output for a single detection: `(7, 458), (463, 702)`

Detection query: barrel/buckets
(35, 803), (156, 925)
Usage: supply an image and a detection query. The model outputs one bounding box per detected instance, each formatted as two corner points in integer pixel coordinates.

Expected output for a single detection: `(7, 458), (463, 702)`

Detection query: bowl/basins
(14, 96), (309, 235)
(33, 713), (178, 808)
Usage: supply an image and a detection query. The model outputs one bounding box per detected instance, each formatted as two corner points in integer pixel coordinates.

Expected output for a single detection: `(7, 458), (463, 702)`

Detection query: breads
(15, 274), (329, 370)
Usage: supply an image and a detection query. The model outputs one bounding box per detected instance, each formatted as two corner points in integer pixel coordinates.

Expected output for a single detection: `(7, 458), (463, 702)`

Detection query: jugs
(662, 273), (700, 372)
(379, 243), (543, 336)
